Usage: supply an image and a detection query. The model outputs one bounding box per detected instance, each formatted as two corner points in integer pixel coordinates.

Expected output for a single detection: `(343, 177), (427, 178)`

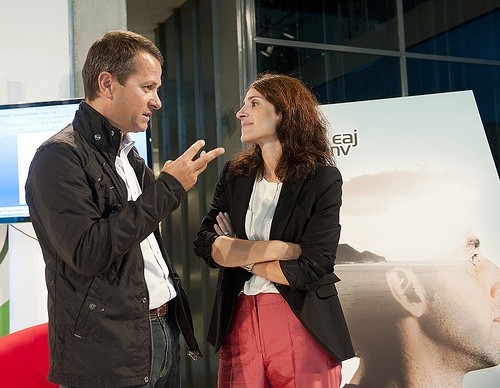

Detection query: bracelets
(245, 263), (255, 273)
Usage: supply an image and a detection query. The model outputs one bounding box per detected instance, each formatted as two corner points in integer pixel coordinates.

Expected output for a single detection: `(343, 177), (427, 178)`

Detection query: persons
(24, 30), (225, 387)
(333, 170), (500, 388)
(192, 73), (356, 388)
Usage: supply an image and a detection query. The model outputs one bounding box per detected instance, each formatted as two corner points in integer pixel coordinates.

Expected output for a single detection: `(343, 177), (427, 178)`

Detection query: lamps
(283, 21), (303, 39)
(261, 43), (275, 57)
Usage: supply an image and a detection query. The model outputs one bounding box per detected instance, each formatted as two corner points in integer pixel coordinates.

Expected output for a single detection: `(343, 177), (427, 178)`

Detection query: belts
(150, 302), (169, 316)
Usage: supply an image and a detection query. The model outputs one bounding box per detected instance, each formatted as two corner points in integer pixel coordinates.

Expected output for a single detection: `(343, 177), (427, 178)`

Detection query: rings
(224, 232), (230, 236)
